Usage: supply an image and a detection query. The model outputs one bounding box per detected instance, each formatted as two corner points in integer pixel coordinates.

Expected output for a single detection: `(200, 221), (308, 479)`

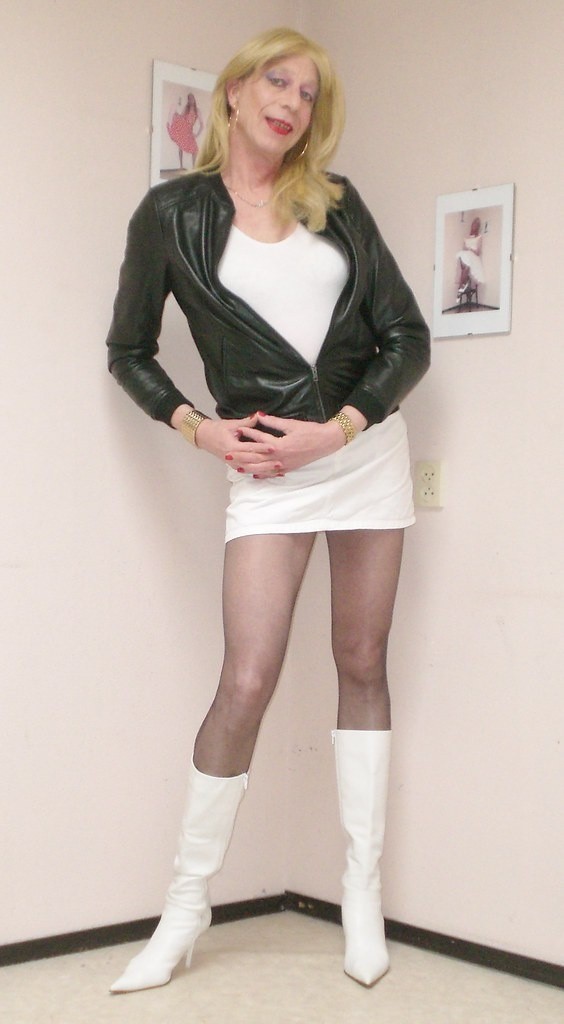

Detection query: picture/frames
(149, 59), (219, 189)
(433, 183), (514, 338)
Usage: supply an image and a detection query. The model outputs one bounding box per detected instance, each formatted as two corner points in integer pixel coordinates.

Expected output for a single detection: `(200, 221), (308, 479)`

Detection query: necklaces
(226, 187), (269, 207)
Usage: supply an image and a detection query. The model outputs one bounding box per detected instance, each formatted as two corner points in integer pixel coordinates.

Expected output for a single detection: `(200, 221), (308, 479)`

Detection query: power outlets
(416, 459), (442, 508)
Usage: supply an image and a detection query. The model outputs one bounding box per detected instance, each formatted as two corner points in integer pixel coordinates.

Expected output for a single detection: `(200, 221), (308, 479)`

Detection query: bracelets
(330, 412), (357, 444)
(180, 410), (212, 450)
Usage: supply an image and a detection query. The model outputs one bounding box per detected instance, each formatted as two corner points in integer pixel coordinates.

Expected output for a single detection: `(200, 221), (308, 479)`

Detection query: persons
(457, 217), (485, 298)
(168, 94), (204, 170)
(107, 27), (430, 985)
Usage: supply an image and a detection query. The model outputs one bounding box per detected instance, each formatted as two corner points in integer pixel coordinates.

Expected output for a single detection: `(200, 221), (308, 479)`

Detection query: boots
(333, 730), (393, 987)
(108, 752), (251, 992)
